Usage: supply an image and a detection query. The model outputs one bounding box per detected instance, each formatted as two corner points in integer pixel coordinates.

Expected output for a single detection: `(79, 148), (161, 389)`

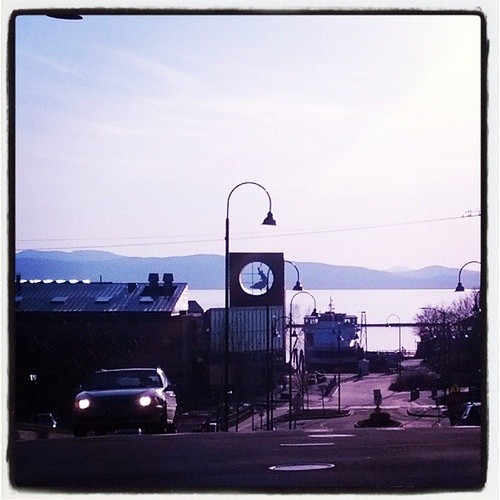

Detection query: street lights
(266, 259), (303, 432)
(289, 291), (320, 432)
(470, 289), (481, 313)
(452, 261), (481, 292)
(337, 322), (361, 415)
(222, 181), (280, 435)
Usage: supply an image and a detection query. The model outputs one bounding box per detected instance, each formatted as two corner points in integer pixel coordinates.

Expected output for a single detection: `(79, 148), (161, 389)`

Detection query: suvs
(455, 402), (482, 427)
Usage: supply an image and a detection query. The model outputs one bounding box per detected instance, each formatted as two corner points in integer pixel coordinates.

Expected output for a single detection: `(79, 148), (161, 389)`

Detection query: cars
(70, 363), (179, 439)
